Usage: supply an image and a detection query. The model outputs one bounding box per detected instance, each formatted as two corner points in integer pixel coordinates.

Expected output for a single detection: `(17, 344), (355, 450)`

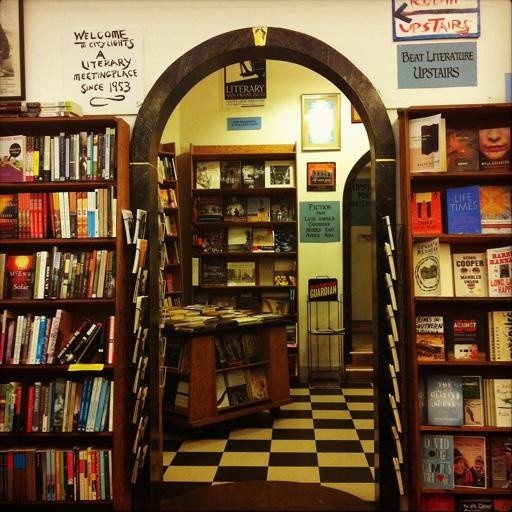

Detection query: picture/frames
(300, 93), (341, 152)
(306, 161), (336, 192)
(351, 102), (363, 124)
(0, 1), (25, 101)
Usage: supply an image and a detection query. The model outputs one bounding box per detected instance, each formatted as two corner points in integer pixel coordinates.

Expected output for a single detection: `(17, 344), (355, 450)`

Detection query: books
(382, 113), (511, 511)
(157, 155), (298, 407)
(0, 100), (148, 502)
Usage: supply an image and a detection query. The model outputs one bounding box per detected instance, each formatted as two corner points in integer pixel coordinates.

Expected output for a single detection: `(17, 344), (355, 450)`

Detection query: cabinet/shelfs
(189, 141), (300, 382)
(306, 275), (344, 390)
(158, 141), (185, 307)
(161, 304), (294, 431)
(0, 115), (129, 511)
(394, 102), (511, 511)
